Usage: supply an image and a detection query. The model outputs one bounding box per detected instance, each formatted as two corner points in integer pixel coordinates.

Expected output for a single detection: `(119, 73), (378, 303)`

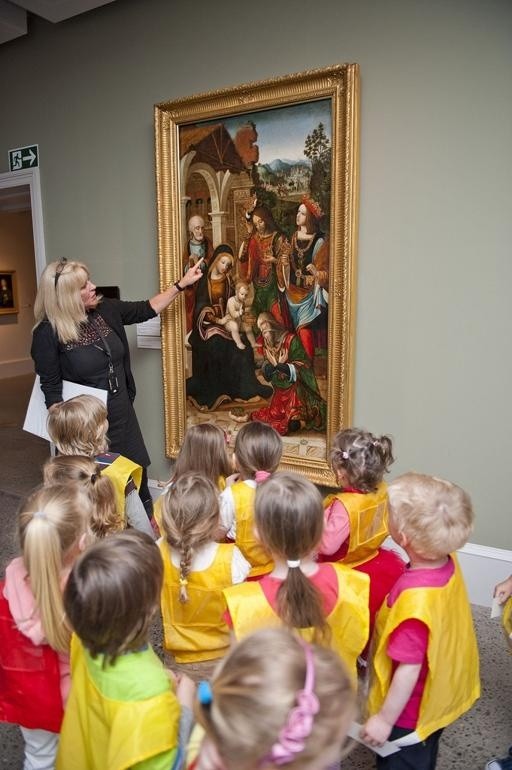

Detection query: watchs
(171, 281), (182, 293)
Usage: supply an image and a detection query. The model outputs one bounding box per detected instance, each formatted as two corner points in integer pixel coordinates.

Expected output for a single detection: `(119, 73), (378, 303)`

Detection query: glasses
(53, 254), (68, 295)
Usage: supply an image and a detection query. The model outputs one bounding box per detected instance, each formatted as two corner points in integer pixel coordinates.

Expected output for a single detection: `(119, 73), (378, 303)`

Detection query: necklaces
(291, 227), (315, 256)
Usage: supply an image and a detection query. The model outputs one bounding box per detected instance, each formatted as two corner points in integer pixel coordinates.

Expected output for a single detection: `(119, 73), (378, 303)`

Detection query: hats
(300, 194), (325, 221)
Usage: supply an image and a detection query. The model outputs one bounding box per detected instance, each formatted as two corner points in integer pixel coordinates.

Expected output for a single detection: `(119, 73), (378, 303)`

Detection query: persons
(42, 454), (126, 539)
(187, 215), (214, 351)
(238, 209), (290, 314)
(29, 256), (203, 539)
(156, 470), (254, 687)
(273, 195), (329, 368)
(223, 470), (370, 694)
(250, 312), (328, 436)
(218, 420), (282, 582)
(187, 625), (354, 770)
(358, 472), (482, 769)
(318, 429), (409, 670)
(1, 484), (90, 770)
(45, 394), (158, 540)
(57, 531), (195, 769)
(186, 244), (273, 411)
(484, 568), (512, 770)
(150, 423), (237, 537)
(225, 281), (263, 351)
